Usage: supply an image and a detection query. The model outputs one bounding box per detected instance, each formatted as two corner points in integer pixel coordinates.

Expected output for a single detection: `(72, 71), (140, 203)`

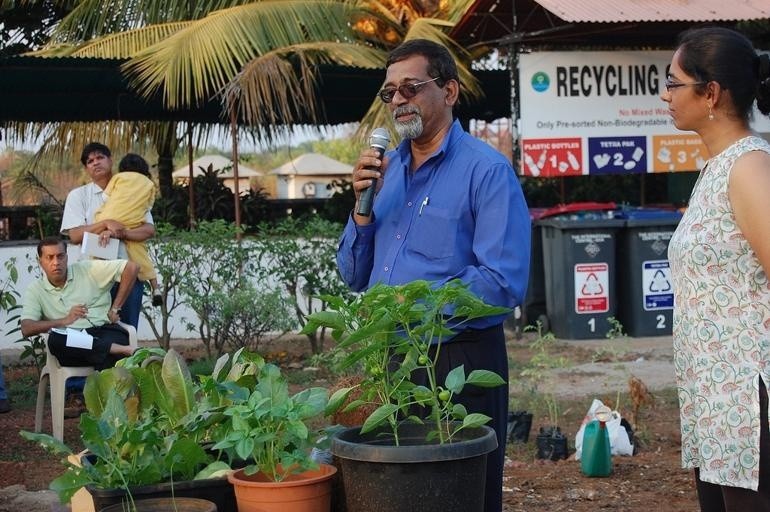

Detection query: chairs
(33, 314), (139, 444)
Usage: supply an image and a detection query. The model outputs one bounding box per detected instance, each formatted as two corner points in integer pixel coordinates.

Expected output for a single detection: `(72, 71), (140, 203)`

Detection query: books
(82, 231), (120, 259)
(52, 327), (94, 350)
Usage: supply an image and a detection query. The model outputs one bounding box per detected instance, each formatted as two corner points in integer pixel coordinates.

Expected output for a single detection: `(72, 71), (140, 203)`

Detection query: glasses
(664, 77), (712, 93)
(379, 75), (441, 104)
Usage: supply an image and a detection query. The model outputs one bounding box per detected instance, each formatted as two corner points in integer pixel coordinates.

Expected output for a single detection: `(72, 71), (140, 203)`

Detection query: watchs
(112, 308), (123, 314)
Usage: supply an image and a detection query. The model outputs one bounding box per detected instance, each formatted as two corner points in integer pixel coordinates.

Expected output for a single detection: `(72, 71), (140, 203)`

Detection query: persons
(60, 142), (155, 333)
(659, 26), (770, 512)
(20, 235), (144, 372)
(337, 38), (531, 512)
(93, 152), (162, 306)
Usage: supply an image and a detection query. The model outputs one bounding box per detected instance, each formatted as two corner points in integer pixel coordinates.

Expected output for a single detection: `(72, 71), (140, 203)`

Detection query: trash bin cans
(501, 202), (682, 340)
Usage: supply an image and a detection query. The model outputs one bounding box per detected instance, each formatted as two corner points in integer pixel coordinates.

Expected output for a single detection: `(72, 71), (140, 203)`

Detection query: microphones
(356, 128), (391, 217)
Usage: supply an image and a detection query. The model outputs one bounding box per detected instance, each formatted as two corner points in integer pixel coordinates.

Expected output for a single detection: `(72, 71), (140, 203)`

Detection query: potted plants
(302, 280), (513, 511)
(512, 318), (574, 462)
(214, 356), (339, 511)
(75, 349), (256, 511)
(20, 413), (219, 511)
